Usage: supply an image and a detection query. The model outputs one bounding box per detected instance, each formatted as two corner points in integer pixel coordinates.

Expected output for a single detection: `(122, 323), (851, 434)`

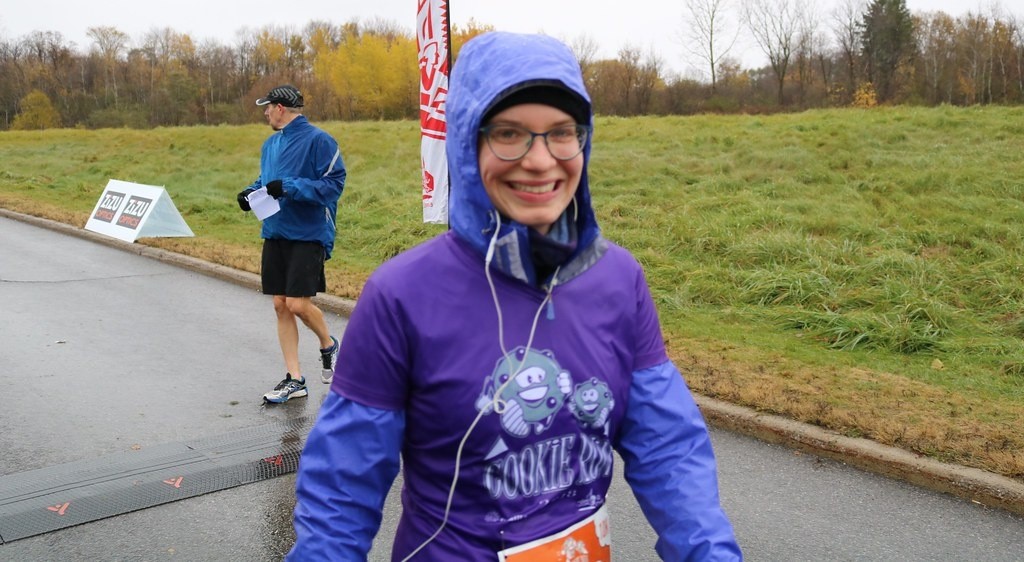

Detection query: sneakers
(318, 336), (340, 383)
(263, 372), (309, 403)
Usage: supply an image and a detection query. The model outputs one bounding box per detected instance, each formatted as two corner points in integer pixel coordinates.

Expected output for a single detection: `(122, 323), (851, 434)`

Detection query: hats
(255, 86), (304, 107)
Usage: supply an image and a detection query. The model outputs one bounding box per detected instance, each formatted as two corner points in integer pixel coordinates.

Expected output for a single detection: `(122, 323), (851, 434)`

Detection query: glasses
(478, 124), (592, 160)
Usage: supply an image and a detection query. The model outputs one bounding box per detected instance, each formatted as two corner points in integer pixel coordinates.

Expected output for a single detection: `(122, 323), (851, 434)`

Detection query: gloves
(266, 180), (284, 200)
(237, 191), (252, 211)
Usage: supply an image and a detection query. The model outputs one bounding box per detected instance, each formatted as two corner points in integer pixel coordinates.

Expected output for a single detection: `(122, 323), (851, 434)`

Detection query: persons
(237, 85), (346, 403)
(285, 31), (743, 562)
